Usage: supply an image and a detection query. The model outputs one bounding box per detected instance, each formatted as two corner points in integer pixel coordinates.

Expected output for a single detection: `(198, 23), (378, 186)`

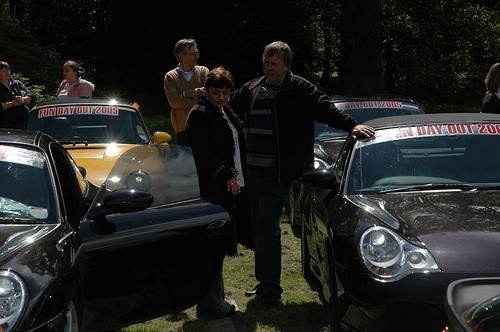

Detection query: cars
(26, 96), (172, 208)
(285, 95), (428, 230)
(0, 129), (234, 332)
(300, 113), (500, 332)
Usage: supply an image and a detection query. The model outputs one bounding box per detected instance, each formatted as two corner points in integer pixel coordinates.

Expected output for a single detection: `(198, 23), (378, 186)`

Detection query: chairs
(364, 144), (403, 176)
(465, 141), (500, 180)
(48, 117), (71, 138)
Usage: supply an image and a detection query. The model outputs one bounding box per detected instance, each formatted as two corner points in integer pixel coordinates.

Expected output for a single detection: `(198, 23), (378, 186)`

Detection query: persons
(0, 62), (36, 130)
(186, 67), (244, 318)
(55, 61), (94, 99)
(229, 41), (374, 303)
(481, 63), (500, 114)
(164, 40), (209, 147)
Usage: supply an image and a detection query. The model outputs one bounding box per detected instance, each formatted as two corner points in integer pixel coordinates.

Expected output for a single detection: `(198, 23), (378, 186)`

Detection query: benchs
(402, 154), (466, 179)
(72, 126), (107, 138)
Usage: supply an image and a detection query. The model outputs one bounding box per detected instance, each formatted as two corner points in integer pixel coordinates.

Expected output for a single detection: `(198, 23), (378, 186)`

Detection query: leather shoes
(245, 284), (283, 311)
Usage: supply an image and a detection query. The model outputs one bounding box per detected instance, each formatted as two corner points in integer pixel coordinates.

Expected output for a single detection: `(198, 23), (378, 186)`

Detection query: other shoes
(195, 301), (236, 320)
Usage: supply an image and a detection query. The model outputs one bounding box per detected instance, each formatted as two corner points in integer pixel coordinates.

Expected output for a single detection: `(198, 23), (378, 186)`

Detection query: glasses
(184, 51), (200, 57)
